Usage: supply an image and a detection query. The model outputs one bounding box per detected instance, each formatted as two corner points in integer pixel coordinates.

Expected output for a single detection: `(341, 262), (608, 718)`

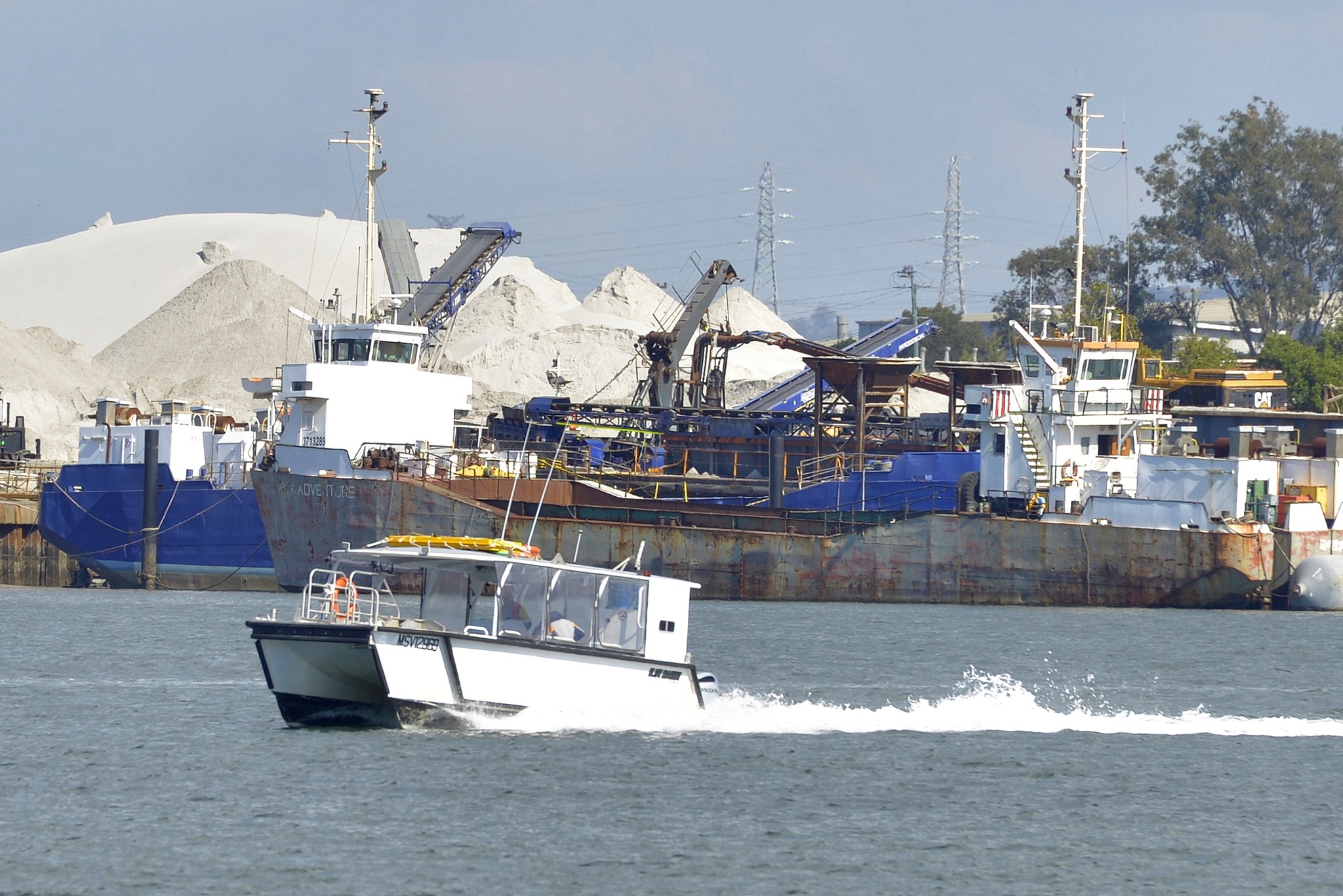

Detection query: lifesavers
(564, 414), (578, 429)
(1061, 459), (1077, 478)
(330, 577), (357, 619)
(512, 551), (541, 560)
(518, 545), (541, 553)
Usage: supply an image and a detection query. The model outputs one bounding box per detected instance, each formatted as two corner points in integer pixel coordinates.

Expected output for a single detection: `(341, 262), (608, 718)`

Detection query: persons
(1026, 493), (1046, 515)
(501, 588), (532, 630)
(548, 607), (585, 642)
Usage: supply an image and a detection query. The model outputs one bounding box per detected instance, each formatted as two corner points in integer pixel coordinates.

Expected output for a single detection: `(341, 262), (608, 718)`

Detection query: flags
(989, 389), (1010, 418)
(1144, 389), (1164, 415)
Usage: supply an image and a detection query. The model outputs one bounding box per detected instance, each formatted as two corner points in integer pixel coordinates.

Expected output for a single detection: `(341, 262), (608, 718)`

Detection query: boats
(39, 84), (1343, 612)
(245, 531), (721, 722)
